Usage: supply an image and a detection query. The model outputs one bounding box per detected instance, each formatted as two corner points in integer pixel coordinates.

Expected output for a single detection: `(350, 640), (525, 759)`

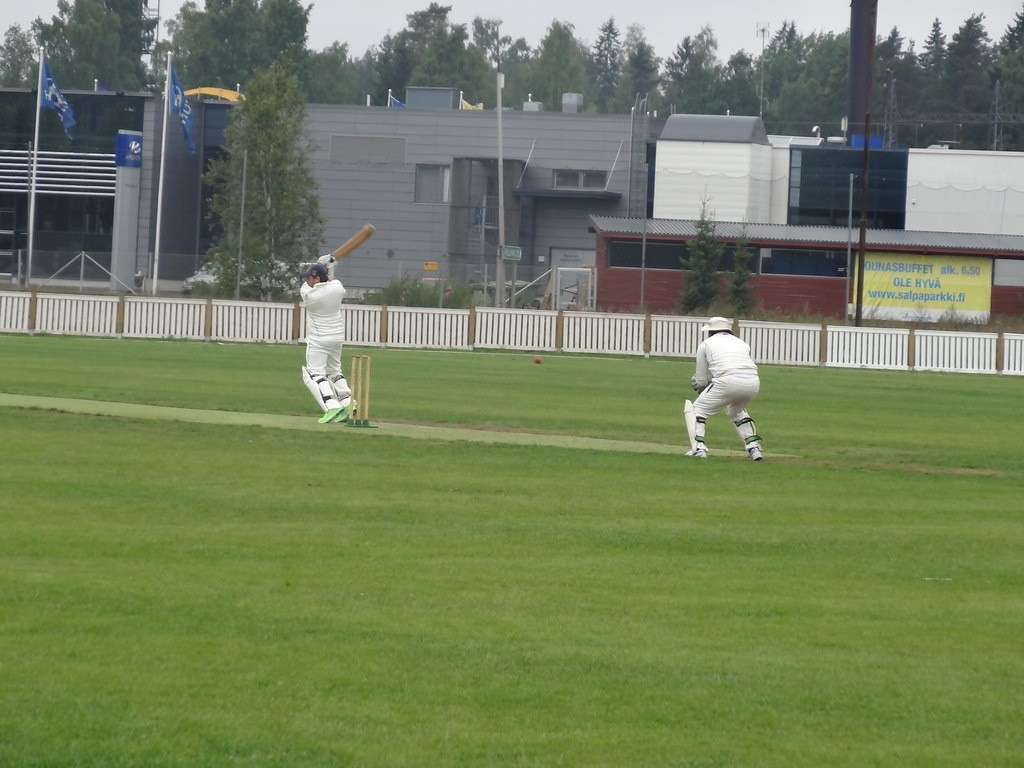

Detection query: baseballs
(534, 356), (541, 363)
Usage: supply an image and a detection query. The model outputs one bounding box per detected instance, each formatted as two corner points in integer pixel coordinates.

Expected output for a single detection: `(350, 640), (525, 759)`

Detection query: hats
(302, 264), (328, 280)
(699, 317), (735, 334)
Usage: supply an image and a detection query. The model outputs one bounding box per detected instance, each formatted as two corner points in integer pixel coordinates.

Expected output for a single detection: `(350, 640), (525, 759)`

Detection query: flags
(40, 53), (78, 142)
(169, 60), (195, 153)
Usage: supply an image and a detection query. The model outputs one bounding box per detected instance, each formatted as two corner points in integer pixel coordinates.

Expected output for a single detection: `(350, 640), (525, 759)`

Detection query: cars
(182, 258), (295, 295)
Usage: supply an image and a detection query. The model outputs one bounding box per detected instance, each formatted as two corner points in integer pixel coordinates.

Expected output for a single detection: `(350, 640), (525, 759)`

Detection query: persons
(298, 255), (358, 424)
(683, 315), (764, 458)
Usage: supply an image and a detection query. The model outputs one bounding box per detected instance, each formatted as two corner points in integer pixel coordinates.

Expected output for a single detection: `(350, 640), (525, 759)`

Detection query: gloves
(315, 254), (337, 274)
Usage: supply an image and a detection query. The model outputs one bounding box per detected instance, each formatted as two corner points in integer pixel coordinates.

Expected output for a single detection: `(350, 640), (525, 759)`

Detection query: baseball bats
(331, 223), (375, 264)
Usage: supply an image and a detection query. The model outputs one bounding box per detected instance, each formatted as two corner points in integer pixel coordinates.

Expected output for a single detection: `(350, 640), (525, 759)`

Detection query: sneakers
(317, 407), (344, 423)
(747, 449), (763, 462)
(685, 447), (709, 458)
(334, 402), (358, 422)
